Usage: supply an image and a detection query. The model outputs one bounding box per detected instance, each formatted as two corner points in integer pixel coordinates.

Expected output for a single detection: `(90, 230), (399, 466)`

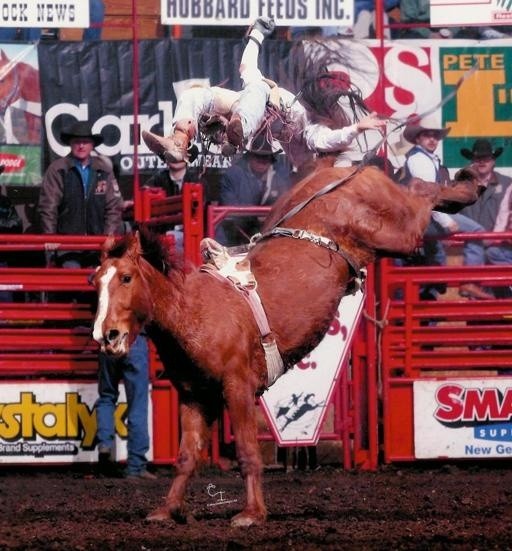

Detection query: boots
(219, 112), (245, 158)
(140, 118), (198, 170)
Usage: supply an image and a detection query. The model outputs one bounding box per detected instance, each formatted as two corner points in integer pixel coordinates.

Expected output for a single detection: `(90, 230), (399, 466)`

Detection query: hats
(459, 139), (505, 162)
(159, 139), (200, 166)
(60, 120), (106, 149)
(403, 118), (452, 146)
(242, 121), (284, 158)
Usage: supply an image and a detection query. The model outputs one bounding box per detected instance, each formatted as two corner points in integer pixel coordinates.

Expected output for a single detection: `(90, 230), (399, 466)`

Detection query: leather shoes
(457, 281), (498, 301)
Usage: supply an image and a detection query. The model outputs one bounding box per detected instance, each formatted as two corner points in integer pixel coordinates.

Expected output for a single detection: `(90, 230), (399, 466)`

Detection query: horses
(87, 37), (489, 530)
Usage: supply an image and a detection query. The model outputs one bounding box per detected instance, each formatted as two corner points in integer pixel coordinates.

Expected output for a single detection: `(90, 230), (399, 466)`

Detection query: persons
(452, 138), (511, 301)
(215, 128), (297, 248)
(31, 122), (139, 305)
(142, 14), (391, 172)
(95, 326), (158, 482)
(136, 162), (238, 273)
(399, 121), (497, 303)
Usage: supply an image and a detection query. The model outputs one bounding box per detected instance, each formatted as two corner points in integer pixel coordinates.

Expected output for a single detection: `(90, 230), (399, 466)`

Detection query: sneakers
(126, 469), (159, 482)
(98, 447), (119, 478)
